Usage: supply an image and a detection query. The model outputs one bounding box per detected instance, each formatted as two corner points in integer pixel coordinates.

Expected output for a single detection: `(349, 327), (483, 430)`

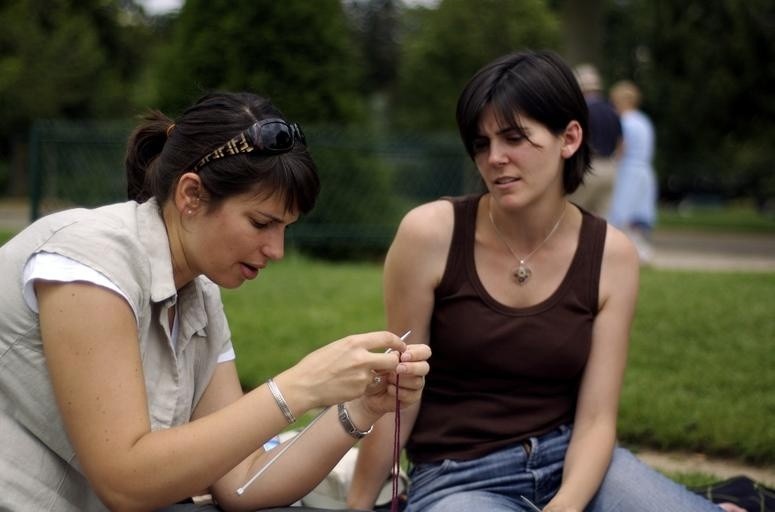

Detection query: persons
(1, 88), (434, 510)
(605, 79), (660, 271)
(561, 57), (625, 220)
(339, 44), (752, 512)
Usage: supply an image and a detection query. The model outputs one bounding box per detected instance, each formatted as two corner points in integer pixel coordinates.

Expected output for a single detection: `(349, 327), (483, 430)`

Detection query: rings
(374, 375), (383, 385)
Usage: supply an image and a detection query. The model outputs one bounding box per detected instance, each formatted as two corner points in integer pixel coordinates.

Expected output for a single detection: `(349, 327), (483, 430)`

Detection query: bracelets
(337, 403), (376, 441)
(267, 377), (296, 425)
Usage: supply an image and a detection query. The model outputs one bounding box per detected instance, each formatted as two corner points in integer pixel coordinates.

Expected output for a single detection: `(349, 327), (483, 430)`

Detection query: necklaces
(487, 193), (570, 287)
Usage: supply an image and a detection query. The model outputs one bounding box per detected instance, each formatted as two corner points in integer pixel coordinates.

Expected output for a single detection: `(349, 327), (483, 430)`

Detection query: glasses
(191, 117), (307, 174)
